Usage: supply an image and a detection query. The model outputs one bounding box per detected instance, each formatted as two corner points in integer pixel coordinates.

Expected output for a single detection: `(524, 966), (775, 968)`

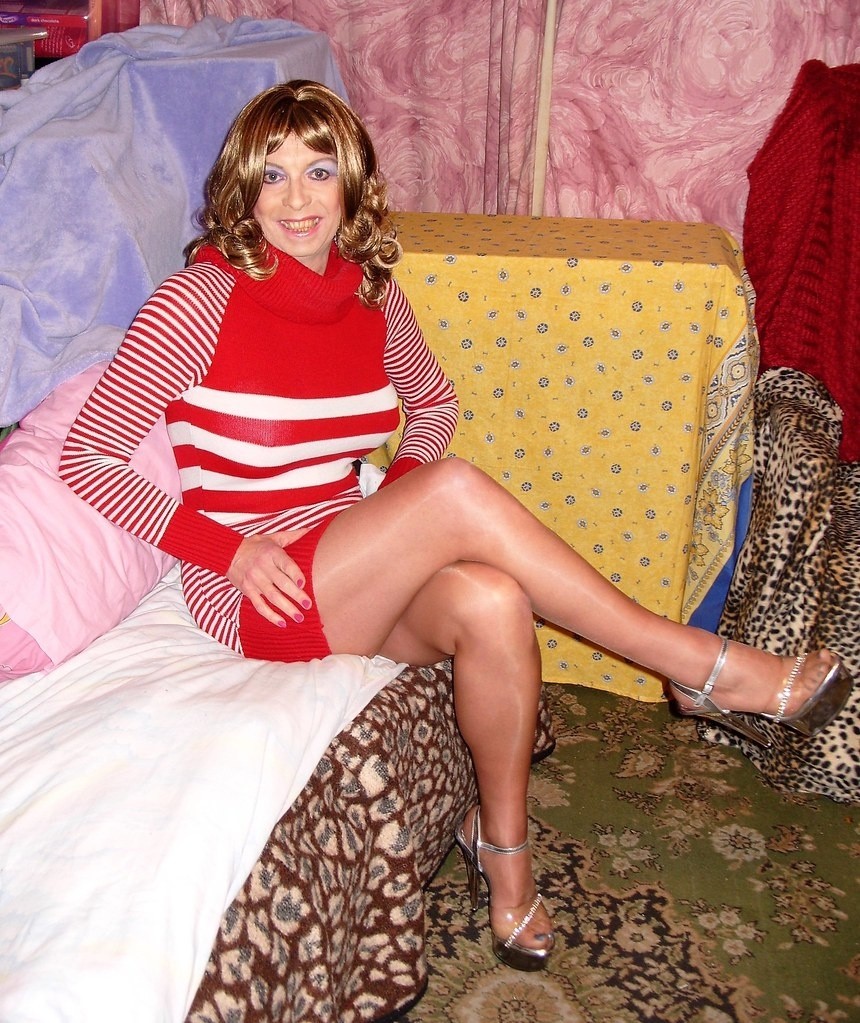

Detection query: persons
(58, 80), (853, 972)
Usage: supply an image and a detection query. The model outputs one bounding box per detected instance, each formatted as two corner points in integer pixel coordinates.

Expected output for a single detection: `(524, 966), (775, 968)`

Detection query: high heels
(671, 637), (854, 750)
(454, 806), (555, 971)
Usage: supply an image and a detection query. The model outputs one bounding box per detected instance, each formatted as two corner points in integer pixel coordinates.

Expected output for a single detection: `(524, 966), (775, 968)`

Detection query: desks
(362, 215), (763, 704)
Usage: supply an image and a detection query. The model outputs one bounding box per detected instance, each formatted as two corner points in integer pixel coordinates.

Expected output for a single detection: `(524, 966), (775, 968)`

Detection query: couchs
(0, 423), (556, 1023)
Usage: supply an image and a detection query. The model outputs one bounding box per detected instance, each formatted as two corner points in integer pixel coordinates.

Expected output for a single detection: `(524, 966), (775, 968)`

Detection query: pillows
(0, 361), (183, 681)
